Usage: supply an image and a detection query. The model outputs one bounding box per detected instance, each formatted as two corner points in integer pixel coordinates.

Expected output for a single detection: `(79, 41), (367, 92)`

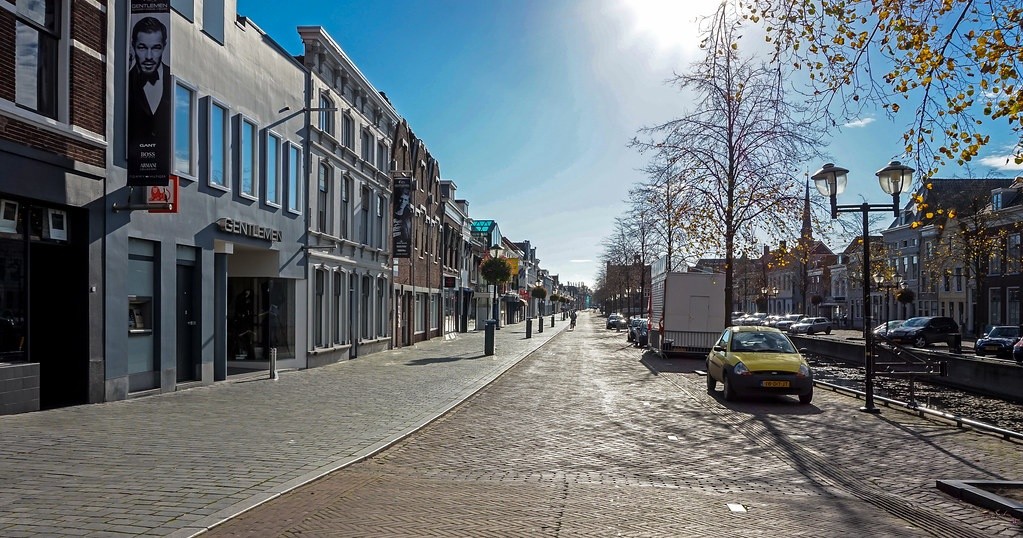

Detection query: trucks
(647, 272), (726, 358)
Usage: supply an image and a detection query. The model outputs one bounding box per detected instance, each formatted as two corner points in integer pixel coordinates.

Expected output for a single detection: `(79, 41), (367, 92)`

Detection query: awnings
(519, 299), (527, 306)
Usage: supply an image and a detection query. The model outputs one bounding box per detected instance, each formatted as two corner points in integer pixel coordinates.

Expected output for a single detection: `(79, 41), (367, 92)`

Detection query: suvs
(745, 312), (769, 326)
(886, 315), (959, 349)
(776, 314), (810, 331)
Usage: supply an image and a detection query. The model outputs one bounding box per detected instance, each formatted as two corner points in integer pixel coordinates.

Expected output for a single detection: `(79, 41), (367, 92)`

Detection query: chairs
(766, 339), (777, 348)
(733, 340), (741, 347)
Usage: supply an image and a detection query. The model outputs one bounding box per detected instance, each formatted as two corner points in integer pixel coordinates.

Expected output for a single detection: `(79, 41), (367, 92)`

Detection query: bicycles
(570, 319), (576, 330)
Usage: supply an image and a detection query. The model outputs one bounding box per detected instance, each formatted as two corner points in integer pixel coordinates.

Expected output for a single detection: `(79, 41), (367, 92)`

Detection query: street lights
(810, 160), (916, 414)
(900, 281), (909, 321)
(535, 279), (542, 317)
(761, 286), (779, 328)
(489, 243), (504, 330)
(872, 270), (903, 344)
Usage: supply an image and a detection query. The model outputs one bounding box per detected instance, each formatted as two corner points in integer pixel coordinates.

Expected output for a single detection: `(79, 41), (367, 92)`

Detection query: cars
(606, 315), (627, 329)
(762, 316), (784, 329)
(704, 326), (815, 403)
(628, 318), (648, 348)
(790, 317), (833, 335)
(1013, 337), (1023, 363)
(975, 325), (1022, 358)
(732, 312), (751, 327)
(873, 319), (906, 340)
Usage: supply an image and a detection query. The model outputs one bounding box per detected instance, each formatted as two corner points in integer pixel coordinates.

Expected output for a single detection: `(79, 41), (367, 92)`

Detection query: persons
(129, 17), (170, 140)
(570, 310), (577, 329)
(393, 188), (409, 219)
